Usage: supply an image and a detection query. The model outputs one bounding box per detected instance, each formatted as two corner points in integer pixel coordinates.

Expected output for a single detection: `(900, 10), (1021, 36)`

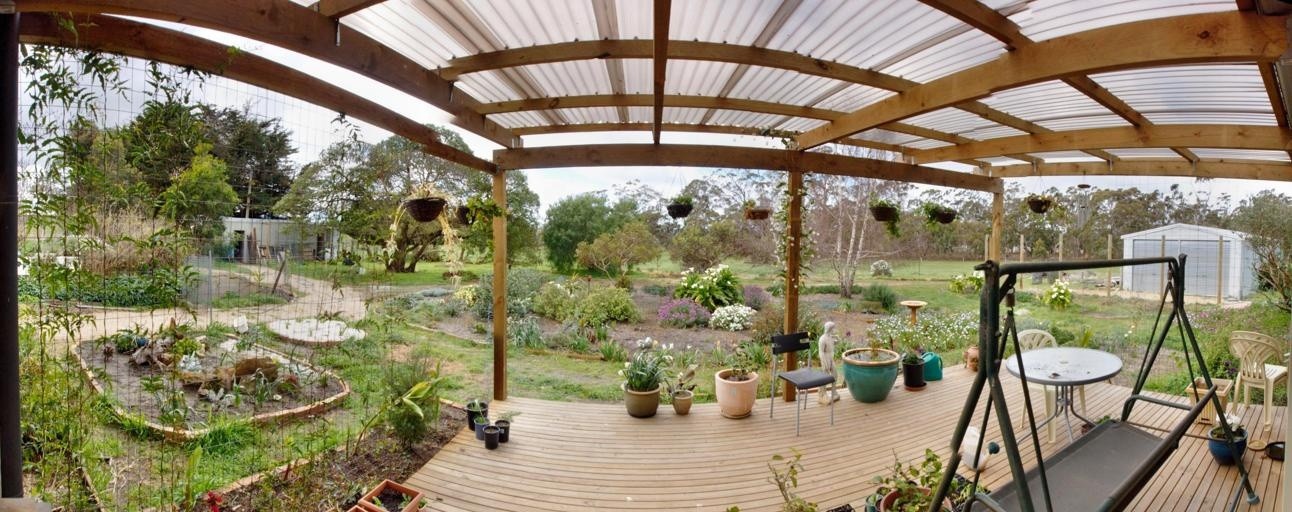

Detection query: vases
(966, 346), (981, 372)
(663, 353), (699, 414)
(619, 382), (661, 418)
(1187, 375), (1234, 426)
(343, 505), (369, 512)
(360, 477), (424, 512)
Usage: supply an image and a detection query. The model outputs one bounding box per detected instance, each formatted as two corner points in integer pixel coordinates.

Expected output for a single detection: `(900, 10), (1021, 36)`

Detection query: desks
(900, 298), (927, 325)
(1006, 349), (1124, 446)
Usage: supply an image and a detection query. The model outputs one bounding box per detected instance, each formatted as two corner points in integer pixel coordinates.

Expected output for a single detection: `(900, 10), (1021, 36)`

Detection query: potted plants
(466, 400), (520, 450)
(711, 342), (773, 418)
(743, 199), (770, 219)
(453, 191), (516, 227)
(1011, 191), (1078, 231)
(841, 327), (900, 404)
(914, 200), (962, 232)
(868, 190), (904, 239)
(864, 446), (984, 512)
(385, 182), (456, 269)
(900, 352), (927, 388)
(1206, 423), (1248, 466)
(666, 195), (694, 218)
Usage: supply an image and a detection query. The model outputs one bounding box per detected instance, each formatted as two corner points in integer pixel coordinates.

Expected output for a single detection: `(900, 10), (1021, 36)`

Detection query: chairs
(769, 331), (835, 435)
(1230, 329), (1291, 426)
(1014, 327), (1088, 444)
(1227, 341), (1290, 433)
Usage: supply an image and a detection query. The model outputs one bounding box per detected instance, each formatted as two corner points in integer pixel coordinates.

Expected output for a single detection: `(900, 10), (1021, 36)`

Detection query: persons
(816, 321), (839, 404)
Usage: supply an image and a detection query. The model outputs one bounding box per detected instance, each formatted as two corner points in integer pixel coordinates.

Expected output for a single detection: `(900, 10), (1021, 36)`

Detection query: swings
(944, 263), (1212, 512)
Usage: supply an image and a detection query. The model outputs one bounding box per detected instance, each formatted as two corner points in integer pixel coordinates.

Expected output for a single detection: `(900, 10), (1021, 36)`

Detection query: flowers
(615, 336), (678, 391)
